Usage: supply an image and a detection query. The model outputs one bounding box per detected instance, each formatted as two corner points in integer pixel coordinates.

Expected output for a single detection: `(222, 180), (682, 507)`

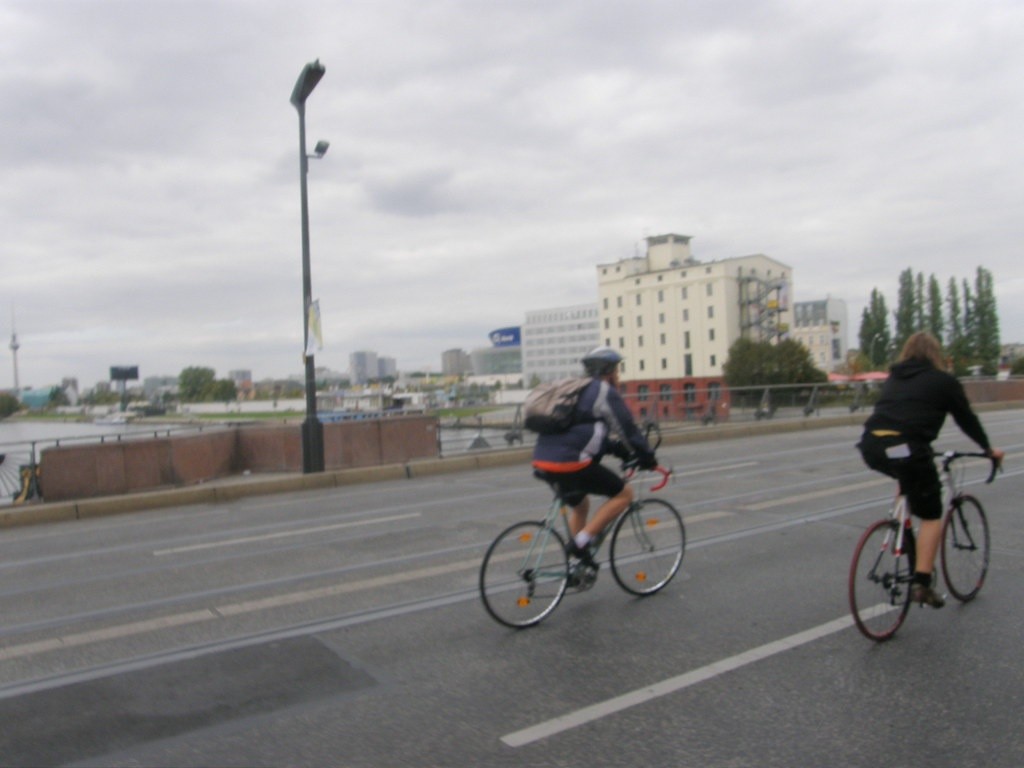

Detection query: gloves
(638, 453), (658, 471)
(612, 440), (630, 462)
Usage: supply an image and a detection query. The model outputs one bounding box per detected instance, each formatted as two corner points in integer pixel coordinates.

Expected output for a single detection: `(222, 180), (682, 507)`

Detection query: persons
(532, 346), (657, 586)
(854, 330), (1003, 609)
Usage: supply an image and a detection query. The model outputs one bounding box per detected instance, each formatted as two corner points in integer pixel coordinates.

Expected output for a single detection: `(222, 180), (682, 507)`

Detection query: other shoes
(910, 583), (945, 609)
(893, 534), (915, 553)
(566, 539), (599, 569)
(567, 573), (595, 587)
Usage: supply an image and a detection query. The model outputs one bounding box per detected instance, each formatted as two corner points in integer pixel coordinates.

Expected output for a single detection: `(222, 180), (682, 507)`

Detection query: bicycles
(845, 453), (1004, 642)
(478, 418), (687, 627)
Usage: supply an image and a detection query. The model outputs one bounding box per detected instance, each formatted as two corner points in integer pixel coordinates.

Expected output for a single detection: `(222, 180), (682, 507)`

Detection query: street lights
(288, 57), (333, 472)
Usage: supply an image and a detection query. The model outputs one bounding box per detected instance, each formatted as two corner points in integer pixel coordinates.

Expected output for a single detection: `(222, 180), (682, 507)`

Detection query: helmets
(583, 347), (622, 371)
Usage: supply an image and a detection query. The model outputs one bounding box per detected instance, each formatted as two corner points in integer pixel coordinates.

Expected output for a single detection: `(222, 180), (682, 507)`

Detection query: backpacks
(524, 374), (604, 433)
